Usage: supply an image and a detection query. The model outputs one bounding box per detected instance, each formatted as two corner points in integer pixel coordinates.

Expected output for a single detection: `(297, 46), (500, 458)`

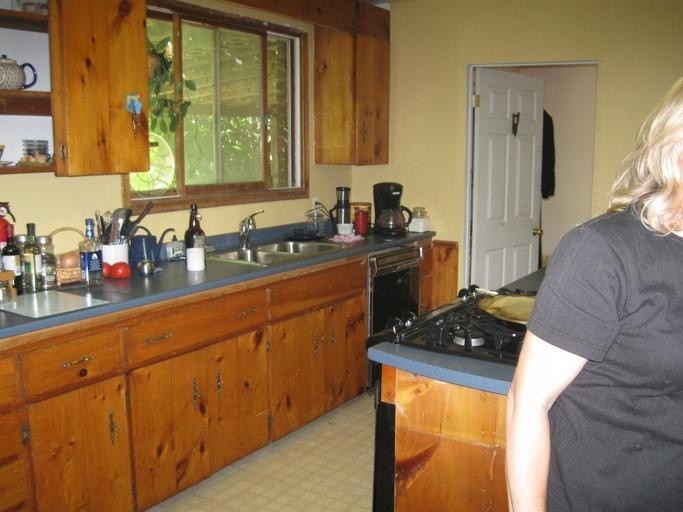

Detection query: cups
(355, 211), (368, 235)
(335, 223), (354, 235)
(185, 247), (204, 273)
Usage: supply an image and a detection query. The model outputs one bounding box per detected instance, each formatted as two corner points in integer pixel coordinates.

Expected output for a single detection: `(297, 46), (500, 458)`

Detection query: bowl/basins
(21, 138), (51, 162)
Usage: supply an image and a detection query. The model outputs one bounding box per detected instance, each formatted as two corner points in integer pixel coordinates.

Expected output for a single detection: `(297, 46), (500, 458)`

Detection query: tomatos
(101, 261), (112, 279)
(109, 260), (131, 280)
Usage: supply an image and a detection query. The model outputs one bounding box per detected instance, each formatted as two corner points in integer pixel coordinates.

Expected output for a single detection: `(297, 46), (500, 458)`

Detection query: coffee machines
(373, 183), (414, 237)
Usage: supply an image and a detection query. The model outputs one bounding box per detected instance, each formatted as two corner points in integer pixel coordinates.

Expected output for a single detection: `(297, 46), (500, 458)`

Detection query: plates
(0, 161), (13, 167)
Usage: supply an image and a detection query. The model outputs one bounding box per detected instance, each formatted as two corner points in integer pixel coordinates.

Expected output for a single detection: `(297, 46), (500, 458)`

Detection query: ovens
(370, 246), (421, 337)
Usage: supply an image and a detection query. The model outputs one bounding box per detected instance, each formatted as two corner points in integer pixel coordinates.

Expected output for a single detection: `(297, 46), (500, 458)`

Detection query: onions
(60, 251), (79, 268)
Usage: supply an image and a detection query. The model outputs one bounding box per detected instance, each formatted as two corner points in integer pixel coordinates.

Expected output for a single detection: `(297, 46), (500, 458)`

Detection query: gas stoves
(385, 284), (535, 366)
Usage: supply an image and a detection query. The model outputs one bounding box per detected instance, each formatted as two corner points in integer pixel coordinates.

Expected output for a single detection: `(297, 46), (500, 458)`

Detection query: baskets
(49, 227), (87, 288)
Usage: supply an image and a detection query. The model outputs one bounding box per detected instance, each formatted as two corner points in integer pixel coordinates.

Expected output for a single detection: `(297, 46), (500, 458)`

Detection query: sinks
(206, 247), (303, 267)
(255, 239), (356, 254)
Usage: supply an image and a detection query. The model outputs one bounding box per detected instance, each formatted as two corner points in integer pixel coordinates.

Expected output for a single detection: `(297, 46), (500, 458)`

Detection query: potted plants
(143, 34), (198, 136)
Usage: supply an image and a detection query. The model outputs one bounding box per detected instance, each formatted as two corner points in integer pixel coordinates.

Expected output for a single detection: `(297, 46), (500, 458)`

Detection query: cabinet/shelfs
(351, 0), (394, 167)
(421, 240), (459, 323)
(119, 285), (269, 511)
(266, 261), (371, 444)
(0, 0), (151, 181)
(0, 320), (135, 512)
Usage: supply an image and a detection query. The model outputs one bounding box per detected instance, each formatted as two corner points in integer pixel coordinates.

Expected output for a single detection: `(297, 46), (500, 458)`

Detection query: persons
(504, 75), (683, 511)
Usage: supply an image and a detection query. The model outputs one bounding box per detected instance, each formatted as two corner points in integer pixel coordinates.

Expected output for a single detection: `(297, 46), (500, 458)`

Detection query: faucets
(238, 209), (264, 250)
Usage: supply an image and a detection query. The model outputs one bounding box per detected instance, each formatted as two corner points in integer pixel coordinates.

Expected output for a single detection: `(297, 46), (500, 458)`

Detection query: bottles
(184, 203), (205, 272)
(80, 217), (103, 286)
(0, 222), (56, 304)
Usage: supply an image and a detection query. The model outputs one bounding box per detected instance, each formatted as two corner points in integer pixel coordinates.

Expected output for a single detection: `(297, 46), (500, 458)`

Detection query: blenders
(330, 185), (350, 234)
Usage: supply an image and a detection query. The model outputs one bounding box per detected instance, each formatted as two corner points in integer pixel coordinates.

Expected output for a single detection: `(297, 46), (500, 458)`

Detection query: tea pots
(0, 54), (36, 90)
(127, 226), (174, 269)
